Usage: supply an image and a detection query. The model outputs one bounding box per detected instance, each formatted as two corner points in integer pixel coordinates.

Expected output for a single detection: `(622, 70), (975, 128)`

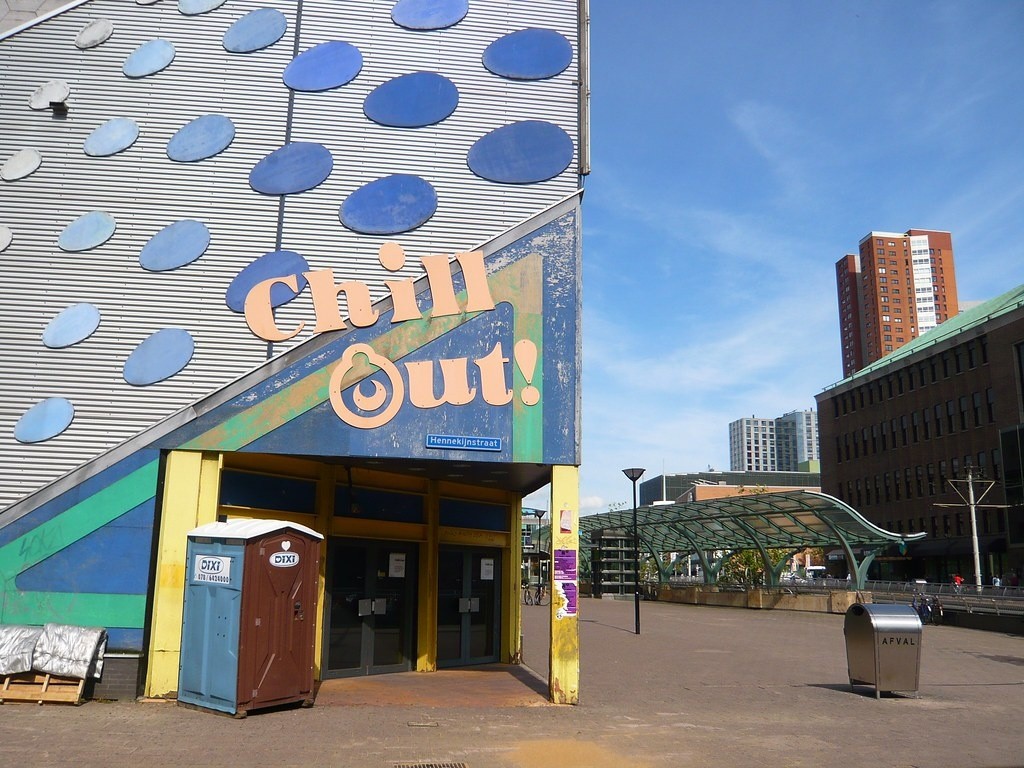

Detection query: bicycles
(520, 580), (551, 606)
(911, 588), (943, 626)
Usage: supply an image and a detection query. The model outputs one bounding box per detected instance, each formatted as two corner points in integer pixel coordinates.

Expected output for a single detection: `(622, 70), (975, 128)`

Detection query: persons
(820, 571), (832, 578)
(1010, 573), (1018, 590)
(953, 574), (962, 590)
(993, 574), (1000, 589)
(790, 572), (798, 586)
(805, 569), (812, 583)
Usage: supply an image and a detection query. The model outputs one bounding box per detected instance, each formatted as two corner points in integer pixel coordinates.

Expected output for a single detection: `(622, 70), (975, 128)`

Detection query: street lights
(534, 509), (546, 605)
(623, 468), (645, 633)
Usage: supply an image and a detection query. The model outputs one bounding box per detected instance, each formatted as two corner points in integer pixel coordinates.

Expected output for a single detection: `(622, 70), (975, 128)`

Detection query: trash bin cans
(843, 603), (923, 700)
